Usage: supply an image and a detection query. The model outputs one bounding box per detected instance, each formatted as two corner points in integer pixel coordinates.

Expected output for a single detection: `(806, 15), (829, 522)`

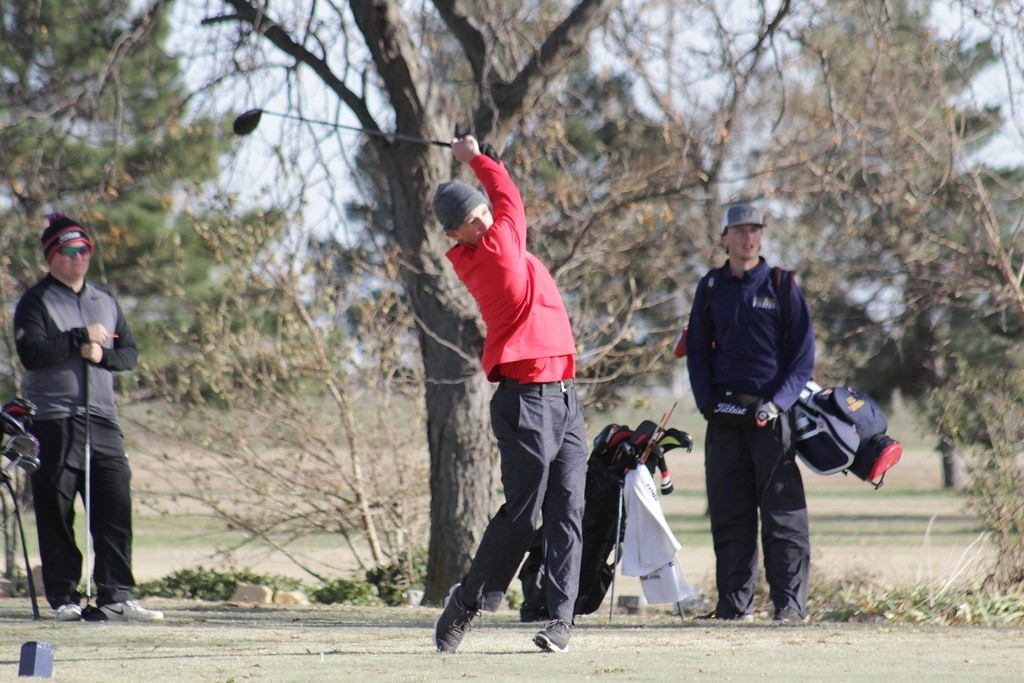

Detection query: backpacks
(671, 265), (902, 491)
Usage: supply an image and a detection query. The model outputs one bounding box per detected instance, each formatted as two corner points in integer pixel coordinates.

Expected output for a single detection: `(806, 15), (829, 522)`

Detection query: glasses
(56, 245), (90, 257)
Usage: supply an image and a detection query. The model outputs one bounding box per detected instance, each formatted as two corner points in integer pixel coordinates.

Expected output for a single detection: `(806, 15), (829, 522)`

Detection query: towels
(621, 462), (696, 607)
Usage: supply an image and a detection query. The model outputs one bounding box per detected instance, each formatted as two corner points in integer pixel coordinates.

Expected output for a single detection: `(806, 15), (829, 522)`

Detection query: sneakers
(692, 609), (753, 623)
(55, 603), (83, 621)
(532, 619), (571, 652)
(97, 600), (163, 623)
(772, 607), (809, 624)
(432, 583), (478, 654)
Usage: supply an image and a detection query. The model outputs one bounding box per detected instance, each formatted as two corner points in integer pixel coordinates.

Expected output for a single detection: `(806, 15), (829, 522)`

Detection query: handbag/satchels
(702, 390), (766, 430)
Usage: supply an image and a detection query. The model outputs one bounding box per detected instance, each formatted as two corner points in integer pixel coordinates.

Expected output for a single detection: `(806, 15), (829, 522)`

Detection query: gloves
(754, 401), (778, 428)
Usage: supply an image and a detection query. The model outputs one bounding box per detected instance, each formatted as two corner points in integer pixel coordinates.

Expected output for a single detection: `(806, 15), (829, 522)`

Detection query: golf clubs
(0, 411), (40, 484)
(233, 109), (452, 148)
(81, 357), (109, 624)
(601, 420), (694, 470)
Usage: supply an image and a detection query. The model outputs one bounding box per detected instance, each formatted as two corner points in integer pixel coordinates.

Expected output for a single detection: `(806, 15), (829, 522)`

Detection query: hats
(433, 180), (489, 230)
(722, 205), (767, 236)
(41, 212), (93, 264)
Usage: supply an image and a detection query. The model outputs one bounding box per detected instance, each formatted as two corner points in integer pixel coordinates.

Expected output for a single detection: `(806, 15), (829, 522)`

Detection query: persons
(14, 211), (164, 623)
(431, 135), (588, 653)
(685, 206), (814, 621)
